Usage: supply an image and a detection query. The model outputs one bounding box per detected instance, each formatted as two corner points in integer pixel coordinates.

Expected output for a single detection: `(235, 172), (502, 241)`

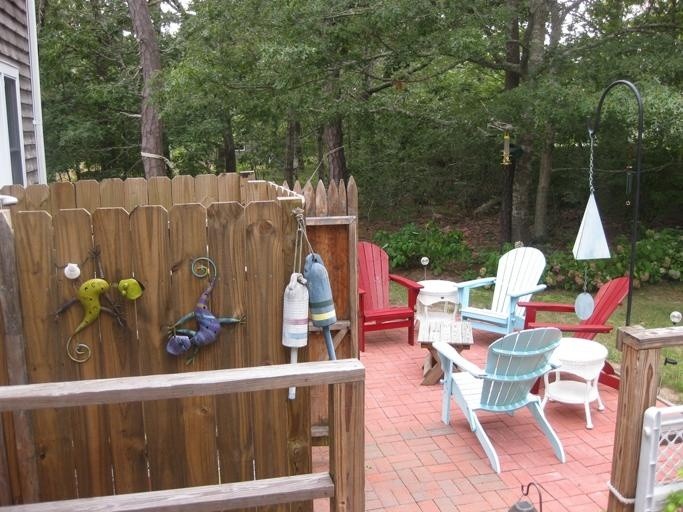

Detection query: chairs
(517, 277), (630, 394)
(453, 246), (547, 337)
(433, 327), (567, 475)
(357, 239), (423, 347)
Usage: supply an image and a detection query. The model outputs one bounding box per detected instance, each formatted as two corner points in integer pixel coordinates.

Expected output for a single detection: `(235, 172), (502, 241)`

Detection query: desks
(416, 279), (460, 317)
(418, 316), (474, 386)
(540, 337), (608, 430)
